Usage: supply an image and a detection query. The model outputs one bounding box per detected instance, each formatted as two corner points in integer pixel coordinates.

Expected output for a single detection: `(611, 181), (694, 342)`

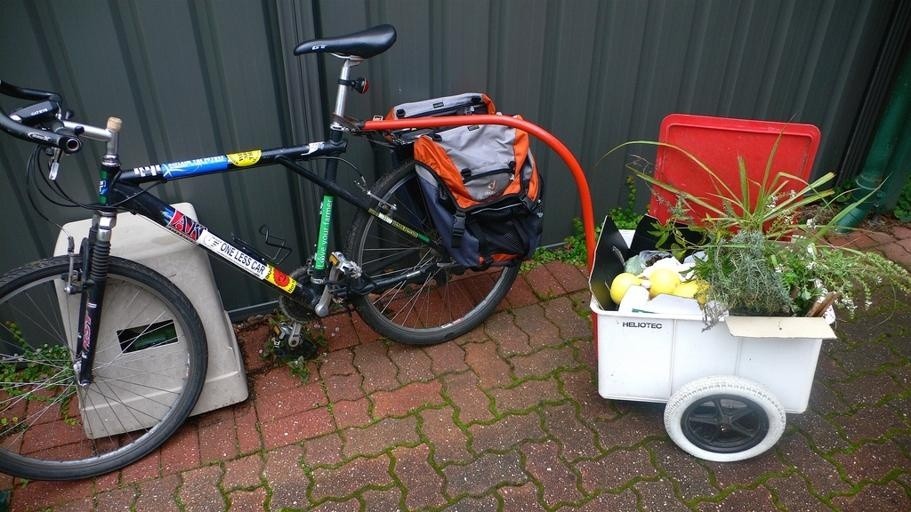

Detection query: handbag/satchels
(413, 115), (545, 273)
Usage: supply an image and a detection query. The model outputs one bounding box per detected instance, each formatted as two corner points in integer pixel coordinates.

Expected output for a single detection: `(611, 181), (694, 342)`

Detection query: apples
(609, 268), (710, 309)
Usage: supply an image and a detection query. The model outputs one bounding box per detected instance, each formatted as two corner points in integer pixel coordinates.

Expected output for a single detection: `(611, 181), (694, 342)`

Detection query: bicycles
(0, 23), (545, 481)
